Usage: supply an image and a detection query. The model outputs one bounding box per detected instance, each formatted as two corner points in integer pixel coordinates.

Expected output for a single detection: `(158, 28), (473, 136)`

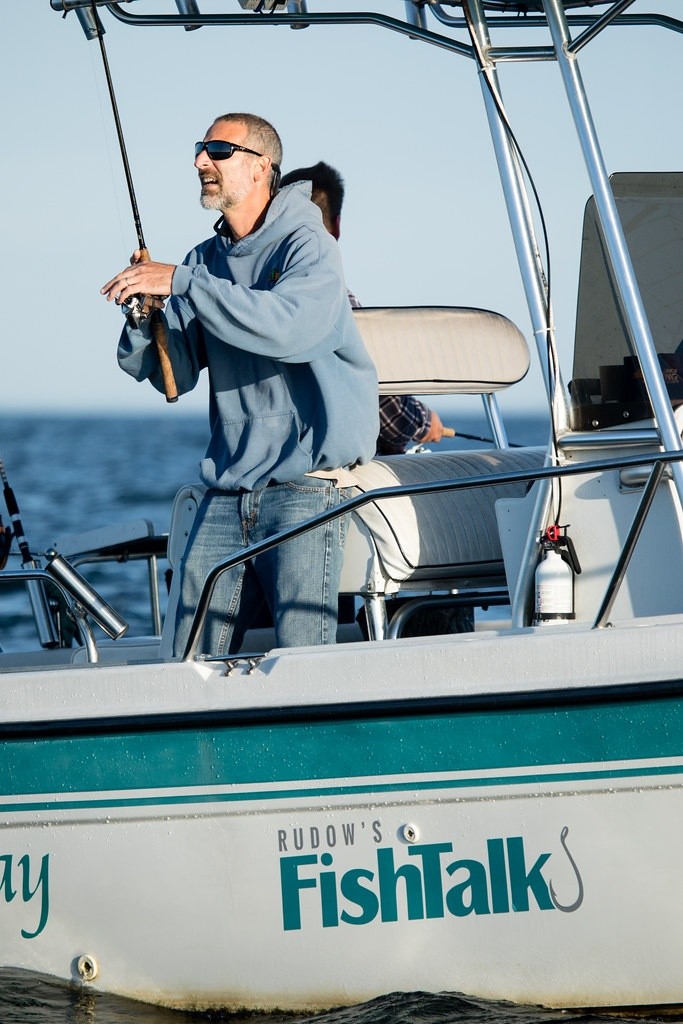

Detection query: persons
(101, 112), (381, 660)
(281, 159), (444, 455)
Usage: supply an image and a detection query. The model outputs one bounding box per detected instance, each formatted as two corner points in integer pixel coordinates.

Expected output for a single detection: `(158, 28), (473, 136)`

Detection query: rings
(125, 277), (133, 288)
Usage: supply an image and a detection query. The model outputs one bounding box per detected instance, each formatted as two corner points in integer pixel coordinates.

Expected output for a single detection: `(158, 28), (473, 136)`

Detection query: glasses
(195, 140), (262, 160)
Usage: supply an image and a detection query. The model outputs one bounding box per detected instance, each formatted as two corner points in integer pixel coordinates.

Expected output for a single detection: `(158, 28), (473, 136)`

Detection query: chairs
(336, 304), (550, 637)
